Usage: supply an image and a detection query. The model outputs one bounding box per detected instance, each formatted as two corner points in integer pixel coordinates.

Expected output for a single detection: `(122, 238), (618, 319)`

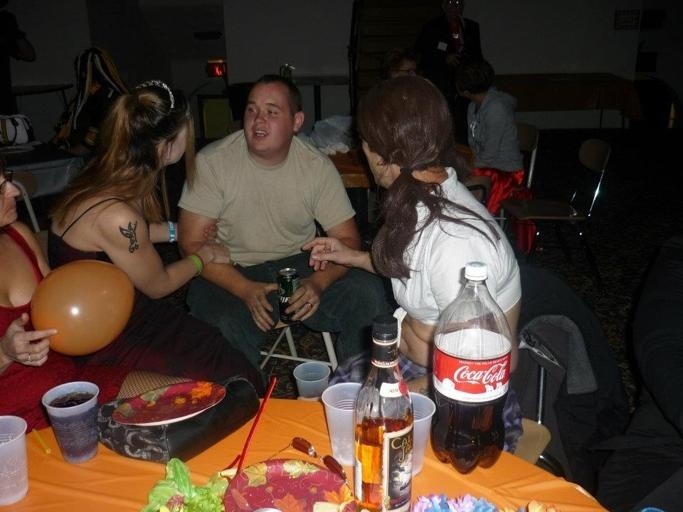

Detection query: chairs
(7, 175), (60, 261)
(488, 123), (539, 231)
(513, 264), (572, 481)
(461, 173), (492, 210)
(507, 136), (612, 294)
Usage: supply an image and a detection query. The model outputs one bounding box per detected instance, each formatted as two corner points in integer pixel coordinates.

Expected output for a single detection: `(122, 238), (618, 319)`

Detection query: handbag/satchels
(0, 114), (36, 145)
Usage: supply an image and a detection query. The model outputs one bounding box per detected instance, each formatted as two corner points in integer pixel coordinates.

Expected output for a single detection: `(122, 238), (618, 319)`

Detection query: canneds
(278, 268), (300, 324)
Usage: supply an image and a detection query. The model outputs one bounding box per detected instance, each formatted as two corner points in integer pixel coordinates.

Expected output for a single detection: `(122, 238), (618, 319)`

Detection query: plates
(224, 459), (356, 511)
(112, 381), (226, 427)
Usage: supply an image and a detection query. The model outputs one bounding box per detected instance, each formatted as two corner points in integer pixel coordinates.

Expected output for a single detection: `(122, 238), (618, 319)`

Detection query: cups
(322, 382), (364, 465)
(42, 380), (99, 463)
(292, 360), (332, 398)
(0, 416), (29, 506)
(408, 392), (436, 475)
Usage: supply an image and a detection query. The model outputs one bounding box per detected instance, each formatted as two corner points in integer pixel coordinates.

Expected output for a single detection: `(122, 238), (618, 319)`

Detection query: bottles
(354, 315), (412, 511)
(431, 260), (514, 475)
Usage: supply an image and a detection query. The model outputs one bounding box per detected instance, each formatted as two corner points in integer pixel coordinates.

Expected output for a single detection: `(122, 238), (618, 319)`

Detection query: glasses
(444, 0), (463, 9)
(0, 169), (13, 195)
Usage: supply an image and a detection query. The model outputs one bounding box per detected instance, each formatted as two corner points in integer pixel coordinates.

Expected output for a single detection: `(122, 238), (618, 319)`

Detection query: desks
(1, 401), (610, 512)
(491, 73), (646, 131)
(324, 142), (471, 222)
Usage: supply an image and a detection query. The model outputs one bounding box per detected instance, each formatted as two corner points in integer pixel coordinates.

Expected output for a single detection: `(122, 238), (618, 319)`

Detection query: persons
(591, 236), (683, 511)
(1, 1), (523, 454)
